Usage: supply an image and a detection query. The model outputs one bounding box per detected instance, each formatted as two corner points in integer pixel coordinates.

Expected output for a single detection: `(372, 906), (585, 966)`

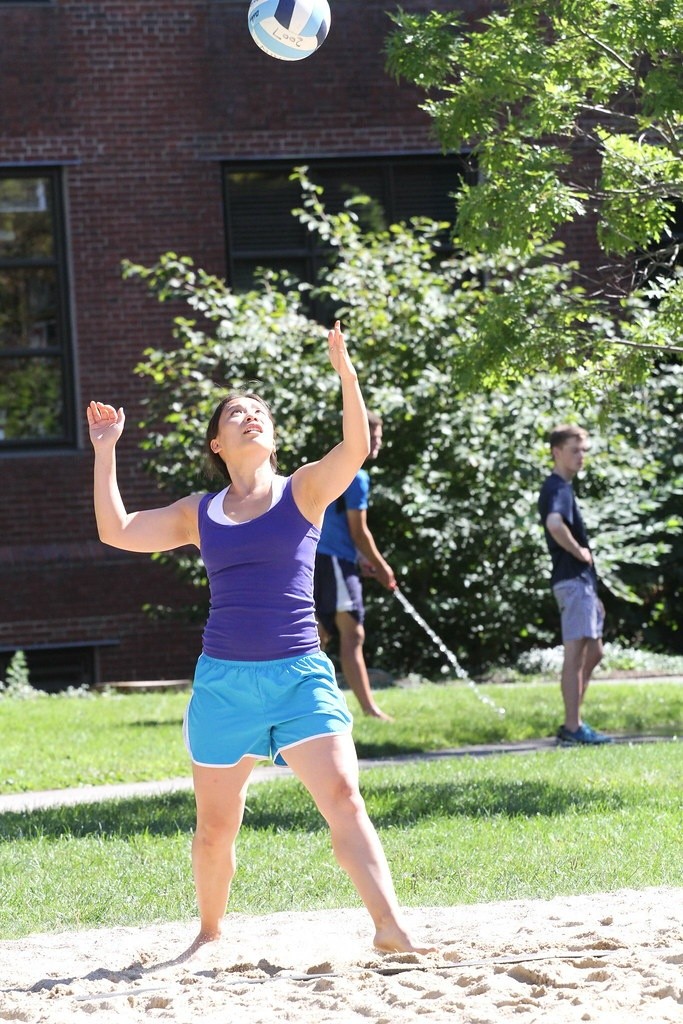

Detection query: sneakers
(557, 723), (614, 748)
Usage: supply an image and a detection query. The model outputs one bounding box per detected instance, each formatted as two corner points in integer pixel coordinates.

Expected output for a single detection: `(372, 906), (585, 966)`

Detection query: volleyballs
(248, 0), (333, 61)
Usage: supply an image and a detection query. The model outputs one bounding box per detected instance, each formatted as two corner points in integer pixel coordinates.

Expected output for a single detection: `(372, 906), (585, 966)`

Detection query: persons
(537, 427), (610, 745)
(86, 317), (416, 954)
(312, 411), (391, 721)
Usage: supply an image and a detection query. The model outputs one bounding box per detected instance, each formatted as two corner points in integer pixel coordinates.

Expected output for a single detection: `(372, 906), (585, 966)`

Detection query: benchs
(0, 639), (121, 683)
